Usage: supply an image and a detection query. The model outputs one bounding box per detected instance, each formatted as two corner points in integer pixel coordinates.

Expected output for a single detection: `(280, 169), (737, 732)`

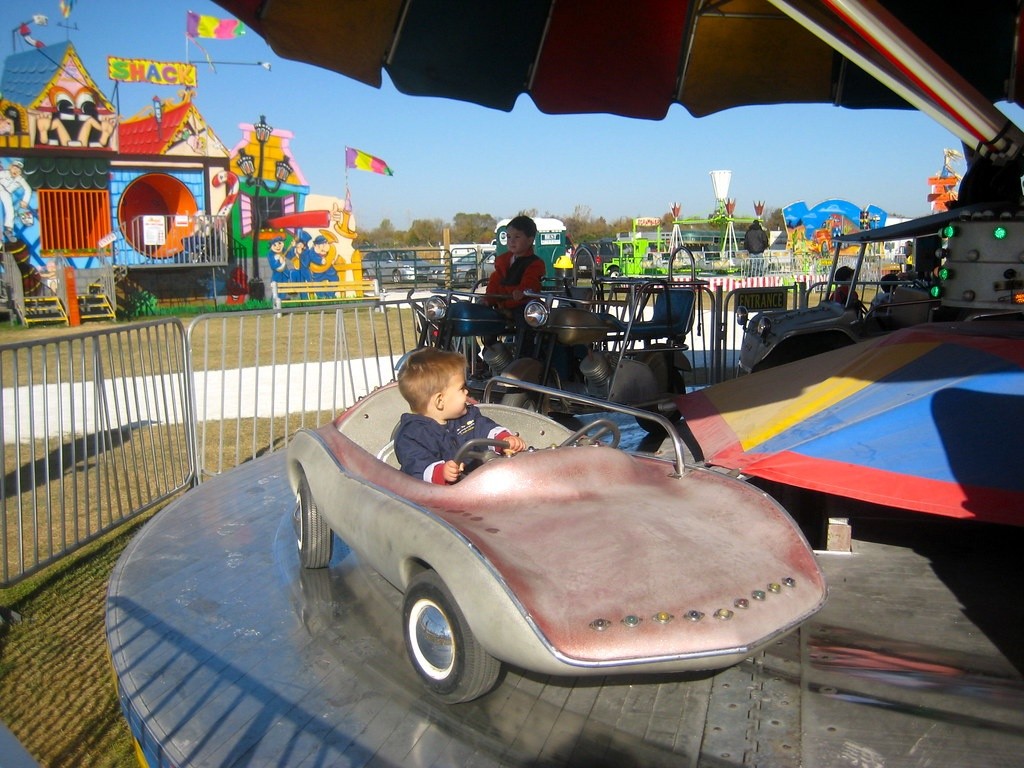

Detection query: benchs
(271, 278), (388, 318)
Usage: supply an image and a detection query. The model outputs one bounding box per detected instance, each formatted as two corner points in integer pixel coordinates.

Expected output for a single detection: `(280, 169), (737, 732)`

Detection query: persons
(869, 273), (899, 311)
(905, 241), (913, 273)
(829, 266), (859, 306)
(476, 215), (545, 360)
(394, 347), (526, 484)
(744, 219), (768, 277)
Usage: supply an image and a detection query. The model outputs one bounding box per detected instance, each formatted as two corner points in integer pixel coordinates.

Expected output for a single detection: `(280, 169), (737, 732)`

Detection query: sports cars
(285, 369), (829, 711)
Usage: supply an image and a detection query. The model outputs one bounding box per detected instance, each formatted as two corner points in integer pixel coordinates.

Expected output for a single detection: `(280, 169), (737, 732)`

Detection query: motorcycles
(416, 249), (708, 411)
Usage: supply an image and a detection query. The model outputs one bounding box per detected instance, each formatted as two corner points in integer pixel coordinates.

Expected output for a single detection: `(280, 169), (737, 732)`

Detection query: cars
(426, 249), (498, 290)
(575, 240), (624, 277)
(361, 248), (433, 285)
(735, 204), (976, 377)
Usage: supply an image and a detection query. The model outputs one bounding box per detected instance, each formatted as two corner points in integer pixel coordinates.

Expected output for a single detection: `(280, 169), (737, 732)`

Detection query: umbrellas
(213, 0), (1024, 176)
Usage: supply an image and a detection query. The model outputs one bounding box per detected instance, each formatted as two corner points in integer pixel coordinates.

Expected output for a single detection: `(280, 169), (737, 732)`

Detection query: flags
(187, 10), (246, 39)
(60, 0), (77, 20)
(346, 147), (394, 176)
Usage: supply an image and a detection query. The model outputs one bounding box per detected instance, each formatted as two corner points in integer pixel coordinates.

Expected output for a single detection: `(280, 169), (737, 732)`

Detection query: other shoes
(471, 367), (490, 380)
